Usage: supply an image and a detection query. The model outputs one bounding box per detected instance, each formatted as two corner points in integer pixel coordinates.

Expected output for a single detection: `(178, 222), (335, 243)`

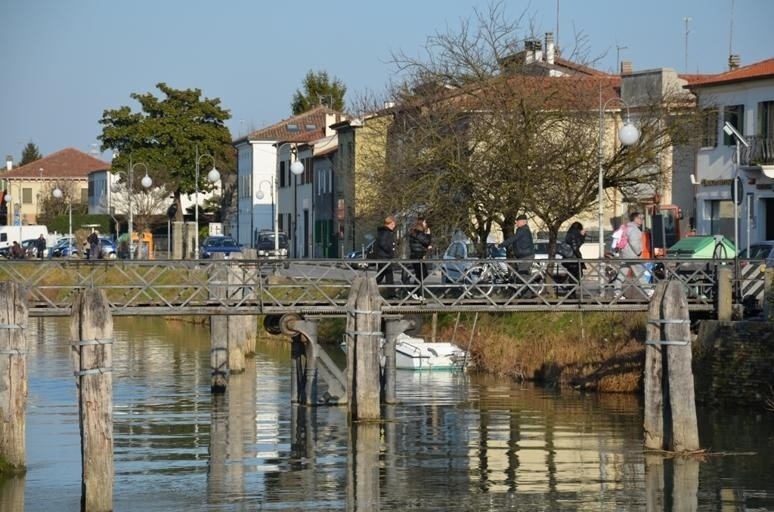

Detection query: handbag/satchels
(558, 243), (574, 257)
(367, 253), (376, 266)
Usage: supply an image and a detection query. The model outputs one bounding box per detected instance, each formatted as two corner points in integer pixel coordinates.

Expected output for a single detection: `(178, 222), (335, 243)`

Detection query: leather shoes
(388, 296), (399, 300)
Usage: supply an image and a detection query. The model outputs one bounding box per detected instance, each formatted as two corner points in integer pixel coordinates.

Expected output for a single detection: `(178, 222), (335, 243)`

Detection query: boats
(340, 332), (471, 371)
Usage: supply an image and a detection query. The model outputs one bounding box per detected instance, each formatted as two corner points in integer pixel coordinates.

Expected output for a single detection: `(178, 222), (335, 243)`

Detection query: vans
(256, 229), (290, 259)
(0, 224), (50, 251)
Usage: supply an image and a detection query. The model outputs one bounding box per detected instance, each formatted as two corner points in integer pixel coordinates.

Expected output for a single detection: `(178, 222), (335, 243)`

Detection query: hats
(517, 215), (527, 220)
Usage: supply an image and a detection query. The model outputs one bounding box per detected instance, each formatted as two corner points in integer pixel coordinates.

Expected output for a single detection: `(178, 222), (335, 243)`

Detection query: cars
(46, 236), (119, 261)
(198, 234), (245, 258)
(348, 235), (437, 270)
(0, 238), (55, 259)
(527, 239), (567, 280)
(439, 240), (508, 289)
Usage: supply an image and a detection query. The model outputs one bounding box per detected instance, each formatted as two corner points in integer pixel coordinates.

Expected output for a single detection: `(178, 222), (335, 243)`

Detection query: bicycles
(462, 243), (546, 299)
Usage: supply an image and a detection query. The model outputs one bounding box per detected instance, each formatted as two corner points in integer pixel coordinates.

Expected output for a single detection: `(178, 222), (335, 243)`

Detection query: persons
(372, 216), (400, 301)
(402, 217), (432, 300)
(12, 241), (25, 258)
(498, 214), (539, 298)
(118, 241), (130, 259)
(613, 211), (655, 300)
(37, 233), (46, 260)
(561, 222), (586, 299)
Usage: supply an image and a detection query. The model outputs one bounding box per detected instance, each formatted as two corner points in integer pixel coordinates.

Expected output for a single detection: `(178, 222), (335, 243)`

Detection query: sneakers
(648, 289), (655, 298)
(408, 291), (426, 300)
(615, 292), (626, 299)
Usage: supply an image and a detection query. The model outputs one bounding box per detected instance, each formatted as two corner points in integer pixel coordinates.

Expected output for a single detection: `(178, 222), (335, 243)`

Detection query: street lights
(597, 78), (639, 299)
(722, 120), (749, 258)
(1, 177), (12, 225)
(110, 153), (152, 259)
(194, 144), (221, 259)
(255, 175), (275, 231)
(274, 140), (305, 251)
(53, 181), (72, 257)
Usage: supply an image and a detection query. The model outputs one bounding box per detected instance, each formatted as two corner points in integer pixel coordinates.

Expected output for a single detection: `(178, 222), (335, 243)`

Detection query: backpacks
(612, 225), (634, 248)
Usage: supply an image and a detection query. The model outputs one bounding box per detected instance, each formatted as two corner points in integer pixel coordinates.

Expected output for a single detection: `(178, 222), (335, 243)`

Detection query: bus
(116, 232), (153, 260)
